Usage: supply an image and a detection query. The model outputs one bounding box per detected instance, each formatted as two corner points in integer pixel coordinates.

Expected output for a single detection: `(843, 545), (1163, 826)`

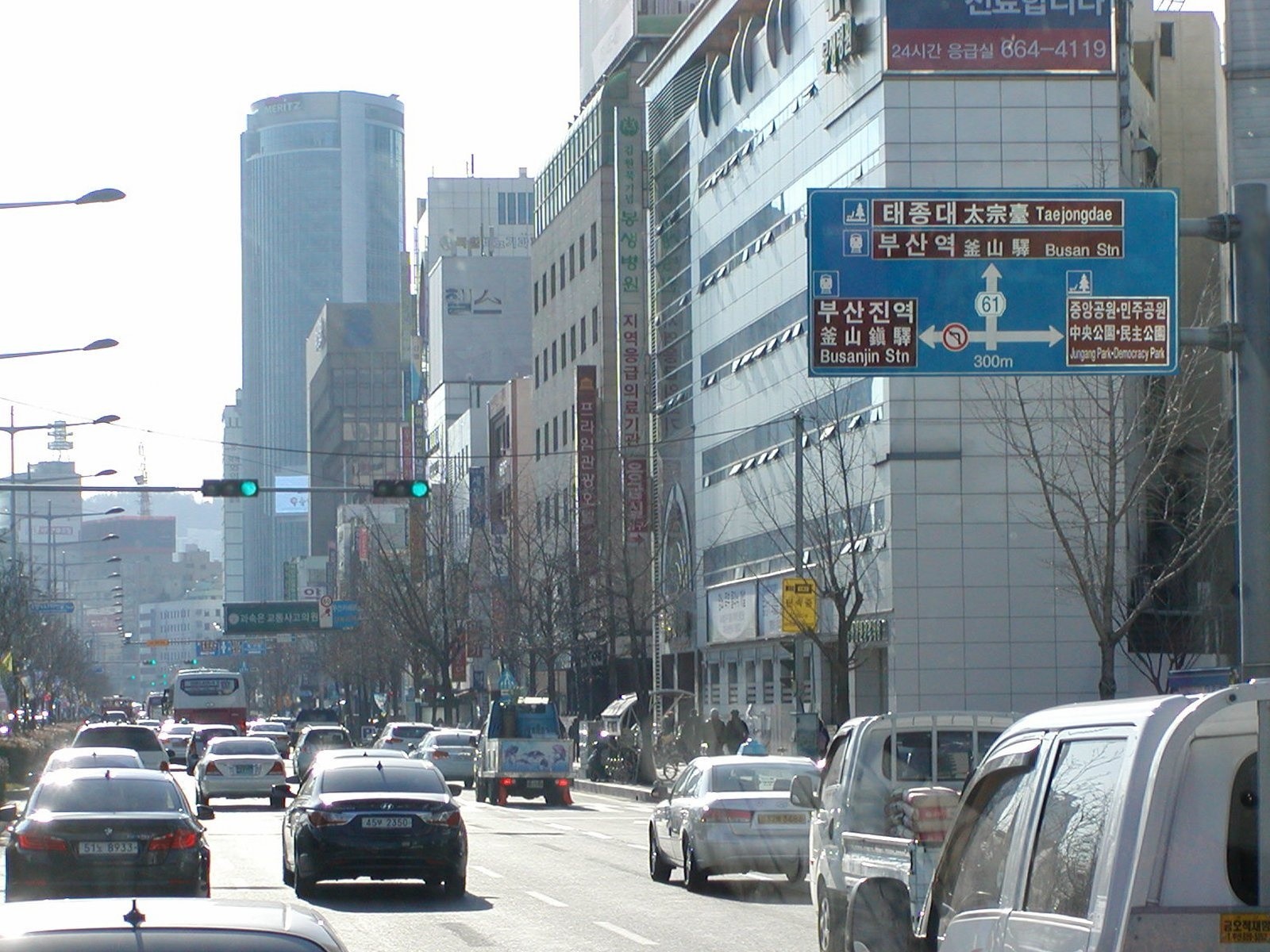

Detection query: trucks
(841, 676), (1270, 952)
(789, 712), (1025, 952)
(468, 696), (576, 806)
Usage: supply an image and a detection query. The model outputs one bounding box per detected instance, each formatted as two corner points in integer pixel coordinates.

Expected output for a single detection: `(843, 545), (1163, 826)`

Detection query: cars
(23, 747), (144, 800)
(90, 706), (482, 810)
(1, 768), (217, 900)
(647, 753), (821, 892)
(272, 756), (469, 899)
(282, 748), (408, 792)
(0, 896), (350, 952)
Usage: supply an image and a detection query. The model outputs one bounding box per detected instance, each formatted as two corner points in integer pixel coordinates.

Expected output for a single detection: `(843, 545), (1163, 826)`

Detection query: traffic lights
(142, 681), (155, 687)
(157, 674), (167, 678)
(142, 660), (156, 665)
(128, 675), (137, 680)
(160, 681), (167, 685)
(373, 478), (432, 498)
(202, 478), (261, 498)
(184, 659), (197, 665)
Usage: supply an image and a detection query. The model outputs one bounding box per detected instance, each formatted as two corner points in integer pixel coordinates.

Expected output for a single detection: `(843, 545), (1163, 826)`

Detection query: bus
(142, 692), (164, 722)
(102, 695), (134, 718)
(162, 668), (247, 736)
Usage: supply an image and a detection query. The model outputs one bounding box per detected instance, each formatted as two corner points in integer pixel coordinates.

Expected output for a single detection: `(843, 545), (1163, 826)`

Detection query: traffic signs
(805, 184), (1182, 376)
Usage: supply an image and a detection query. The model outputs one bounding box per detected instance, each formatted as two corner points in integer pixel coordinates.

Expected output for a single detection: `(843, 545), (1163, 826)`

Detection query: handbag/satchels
(660, 732), (674, 745)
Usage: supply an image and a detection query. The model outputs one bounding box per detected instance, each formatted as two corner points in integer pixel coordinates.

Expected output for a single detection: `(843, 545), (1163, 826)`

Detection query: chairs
(773, 778), (791, 791)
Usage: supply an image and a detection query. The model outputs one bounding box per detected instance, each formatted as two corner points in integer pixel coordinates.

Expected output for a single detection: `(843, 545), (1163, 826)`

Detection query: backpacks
(818, 727), (828, 751)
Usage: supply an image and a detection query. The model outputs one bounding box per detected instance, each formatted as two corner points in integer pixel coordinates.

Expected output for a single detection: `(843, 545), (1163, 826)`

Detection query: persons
(661, 707), (749, 765)
(568, 718), (580, 763)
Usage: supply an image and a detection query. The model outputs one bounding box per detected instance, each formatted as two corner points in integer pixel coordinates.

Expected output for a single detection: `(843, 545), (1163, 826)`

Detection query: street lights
(0, 405), (126, 726)
